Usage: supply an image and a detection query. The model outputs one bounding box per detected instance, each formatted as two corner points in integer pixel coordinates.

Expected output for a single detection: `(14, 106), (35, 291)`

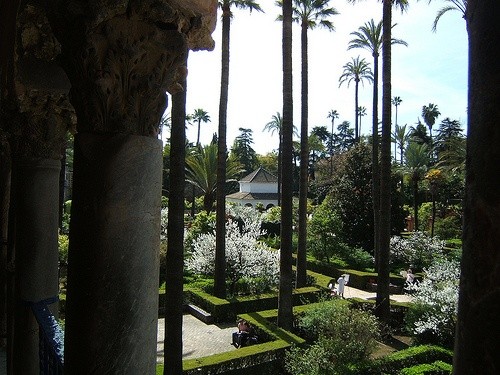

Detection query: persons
(230, 318), (258, 348)
(337, 275), (348, 299)
(406, 269), (413, 297)
(327, 278), (337, 298)
(399, 268), (407, 283)
